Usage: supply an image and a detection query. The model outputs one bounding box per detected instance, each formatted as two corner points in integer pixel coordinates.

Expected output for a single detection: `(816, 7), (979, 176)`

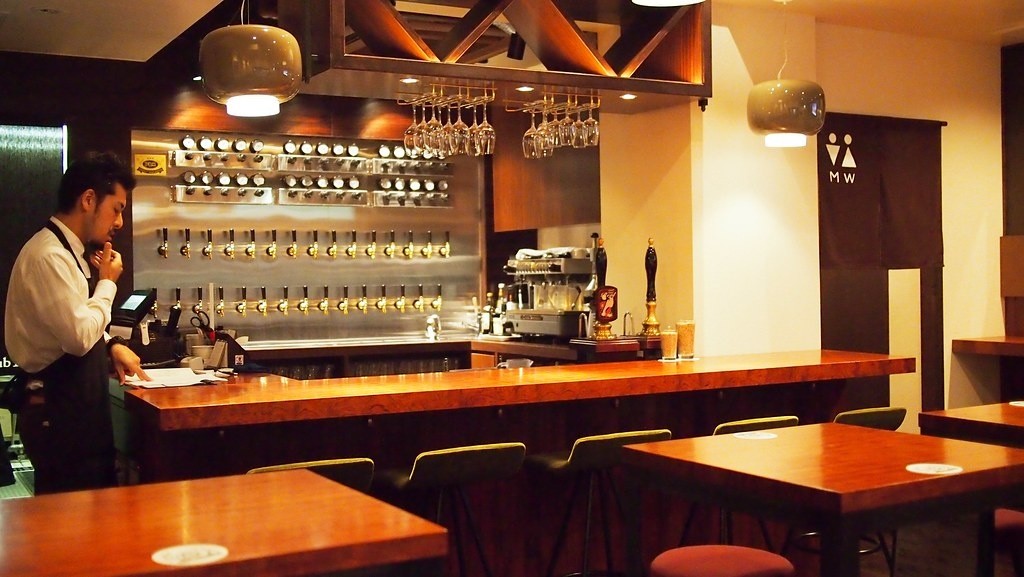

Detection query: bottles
(481, 279), (529, 336)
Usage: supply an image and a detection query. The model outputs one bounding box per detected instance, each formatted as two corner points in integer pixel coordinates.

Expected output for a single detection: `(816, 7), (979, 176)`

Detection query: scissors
(190, 311), (211, 331)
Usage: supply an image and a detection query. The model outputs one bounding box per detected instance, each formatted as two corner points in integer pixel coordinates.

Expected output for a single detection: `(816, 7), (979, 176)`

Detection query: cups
(191, 345), (215, 360)
(675, 320), (696, 359)
(658, 321), (678, 360)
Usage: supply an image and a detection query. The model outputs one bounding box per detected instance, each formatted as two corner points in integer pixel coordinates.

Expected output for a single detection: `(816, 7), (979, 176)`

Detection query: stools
(374, 441), (527, 577)
(649, 545), (795, 577)
(245, 457), (374, 492)
(677, 415), (798, 551)
(525, 429), (672, 577)
(779, 406), (907, 577)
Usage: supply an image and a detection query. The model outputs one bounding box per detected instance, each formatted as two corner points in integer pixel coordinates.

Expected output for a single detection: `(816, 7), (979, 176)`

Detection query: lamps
(198, 0), (302, 117)
(747, 0), (826, 147)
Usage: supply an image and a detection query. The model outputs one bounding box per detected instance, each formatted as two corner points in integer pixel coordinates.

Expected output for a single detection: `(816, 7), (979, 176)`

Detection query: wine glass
(400, 93), (496, 159)
(521, 102), (600, 159)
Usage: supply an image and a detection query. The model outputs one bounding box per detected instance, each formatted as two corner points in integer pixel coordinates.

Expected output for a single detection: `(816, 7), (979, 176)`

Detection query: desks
(919, 401), (1024, 449)
(0, 469), (449, 577)
(621, 422), (1024, 577)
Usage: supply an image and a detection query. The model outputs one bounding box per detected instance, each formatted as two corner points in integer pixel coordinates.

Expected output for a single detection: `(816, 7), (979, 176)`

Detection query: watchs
(106, 336), (128, 354)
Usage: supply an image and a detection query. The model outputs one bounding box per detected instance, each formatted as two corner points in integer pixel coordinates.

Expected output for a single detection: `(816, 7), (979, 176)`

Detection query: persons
(5, 150), (152, 496)
